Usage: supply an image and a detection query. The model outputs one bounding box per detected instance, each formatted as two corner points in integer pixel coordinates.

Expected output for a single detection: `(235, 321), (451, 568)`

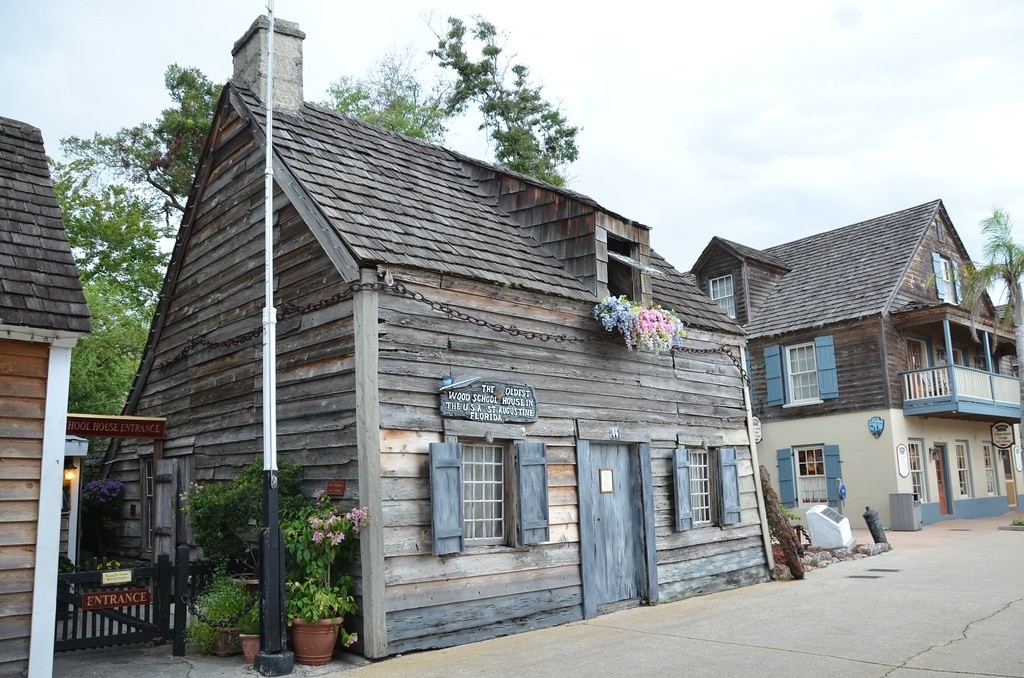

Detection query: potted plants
(179, 456), (309, 665)
(56, 555), (77, 620)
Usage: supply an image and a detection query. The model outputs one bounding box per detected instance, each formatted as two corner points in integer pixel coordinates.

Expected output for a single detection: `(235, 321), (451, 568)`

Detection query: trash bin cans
(888, 493), (923, 532)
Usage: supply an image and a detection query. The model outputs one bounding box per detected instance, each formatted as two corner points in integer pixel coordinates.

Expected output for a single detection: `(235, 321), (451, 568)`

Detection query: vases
(289, 616), (344, 664)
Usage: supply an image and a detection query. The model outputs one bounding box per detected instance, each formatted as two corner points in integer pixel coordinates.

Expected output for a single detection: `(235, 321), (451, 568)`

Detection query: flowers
(82, 479), (123, 511)
(95, 560), (120, 571)
(278, 490), (370, 647)
(595, 295), (687, 352)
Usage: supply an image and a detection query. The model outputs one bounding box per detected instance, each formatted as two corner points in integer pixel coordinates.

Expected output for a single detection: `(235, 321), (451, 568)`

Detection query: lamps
(929, 448), (939, 461)
(937, 348), (947, 361)
(974, 356), (984, 368)
(998, 449), (1007, 459)
(65, 456), (78, 480)
